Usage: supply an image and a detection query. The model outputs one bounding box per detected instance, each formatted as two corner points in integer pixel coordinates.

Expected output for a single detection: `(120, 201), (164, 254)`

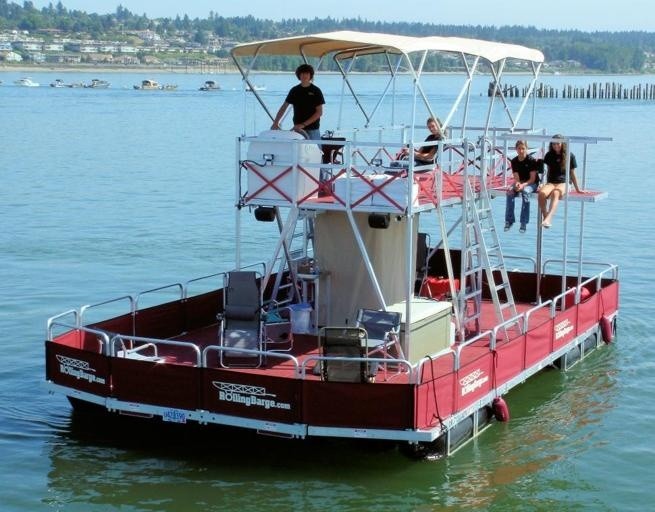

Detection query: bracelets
(302, 124), (306, 127)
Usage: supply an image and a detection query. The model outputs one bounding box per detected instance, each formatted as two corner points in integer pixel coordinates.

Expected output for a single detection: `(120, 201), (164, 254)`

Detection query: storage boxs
(378, 297), (453, 372)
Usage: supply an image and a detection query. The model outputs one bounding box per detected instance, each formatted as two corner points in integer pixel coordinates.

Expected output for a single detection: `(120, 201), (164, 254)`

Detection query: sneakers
(504, 221), (512, 232)
(519, 223), (526, 232)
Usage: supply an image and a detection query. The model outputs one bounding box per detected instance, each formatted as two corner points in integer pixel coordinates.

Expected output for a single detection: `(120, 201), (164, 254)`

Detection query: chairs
(401, 130), (445, 173)
(415, 233), (433, 299)
(313, 327), (378, 383)
(87, 325), (165, 364)
(262, 299), (294, 352)
(217, 271), (264, 369)
(355, 308), (402, 380)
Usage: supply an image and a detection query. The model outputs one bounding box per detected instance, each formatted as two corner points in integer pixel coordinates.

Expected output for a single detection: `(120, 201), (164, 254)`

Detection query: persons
(398, 117), (441, 166)
(504, 141), (539, 232)
(269, 64), (325, 157)
(533, 134), (591, 229)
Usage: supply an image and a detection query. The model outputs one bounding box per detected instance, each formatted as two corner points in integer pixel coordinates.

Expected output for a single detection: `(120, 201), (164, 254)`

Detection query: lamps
(254, 205), (275, 221)
(368, 213), (390, 228)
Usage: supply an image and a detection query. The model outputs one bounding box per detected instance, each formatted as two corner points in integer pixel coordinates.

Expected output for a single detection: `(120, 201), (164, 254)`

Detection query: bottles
(309, 259), (321, 276)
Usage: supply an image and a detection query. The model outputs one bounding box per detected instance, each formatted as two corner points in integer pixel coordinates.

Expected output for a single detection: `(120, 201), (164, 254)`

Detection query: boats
(42, 31), (619, 461)
(0, 75), (267, 93)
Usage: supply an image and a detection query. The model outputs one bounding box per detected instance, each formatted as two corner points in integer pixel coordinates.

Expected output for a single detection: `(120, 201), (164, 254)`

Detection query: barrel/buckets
(422, 276), (459, 300)
(565, 285), (590, 309)
